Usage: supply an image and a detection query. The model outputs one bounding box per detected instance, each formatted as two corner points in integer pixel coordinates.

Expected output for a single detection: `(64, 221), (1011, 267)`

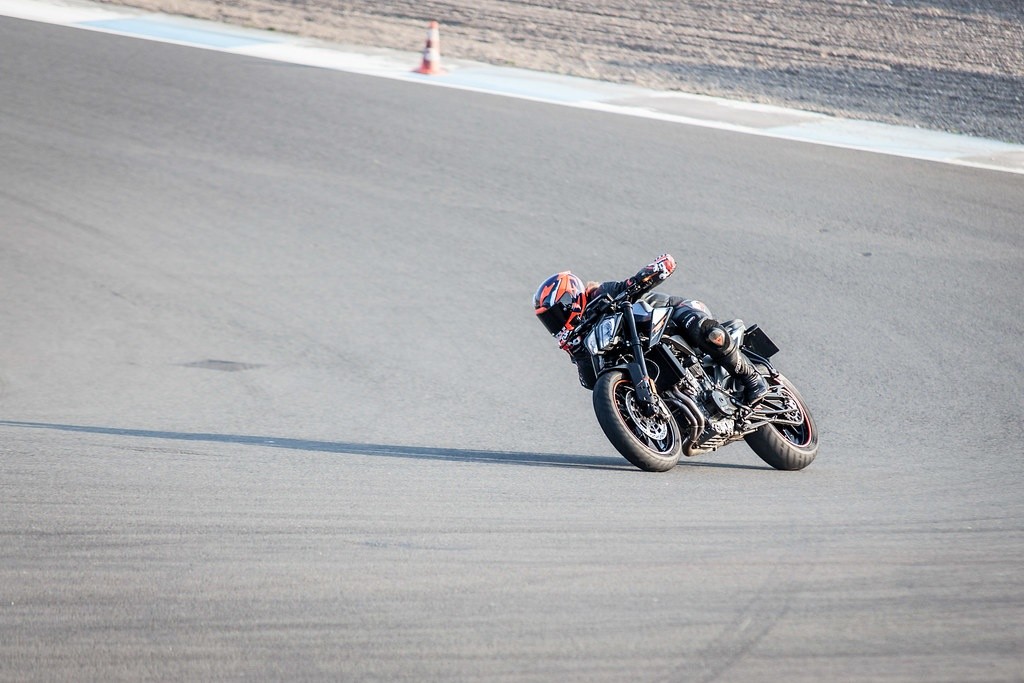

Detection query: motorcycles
(558, 267), (821, 473)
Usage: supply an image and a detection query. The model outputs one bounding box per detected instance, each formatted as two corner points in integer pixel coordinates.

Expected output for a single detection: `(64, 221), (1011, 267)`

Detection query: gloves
(624, 264), (655, 297)
(558, 329), (591, 364)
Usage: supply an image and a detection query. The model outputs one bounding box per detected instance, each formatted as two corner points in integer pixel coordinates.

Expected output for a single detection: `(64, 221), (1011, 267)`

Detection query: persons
(534, 254), (770, 399)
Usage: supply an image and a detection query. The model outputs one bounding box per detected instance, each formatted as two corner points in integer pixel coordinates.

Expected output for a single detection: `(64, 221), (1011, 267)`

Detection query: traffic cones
(413, 20), (447, 75)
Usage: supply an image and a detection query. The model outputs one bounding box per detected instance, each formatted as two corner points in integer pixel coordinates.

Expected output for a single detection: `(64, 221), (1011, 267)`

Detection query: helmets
(533, 271), (587, 340)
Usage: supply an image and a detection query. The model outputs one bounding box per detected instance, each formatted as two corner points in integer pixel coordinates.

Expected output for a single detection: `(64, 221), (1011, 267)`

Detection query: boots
(711, 334), (769, 405)
